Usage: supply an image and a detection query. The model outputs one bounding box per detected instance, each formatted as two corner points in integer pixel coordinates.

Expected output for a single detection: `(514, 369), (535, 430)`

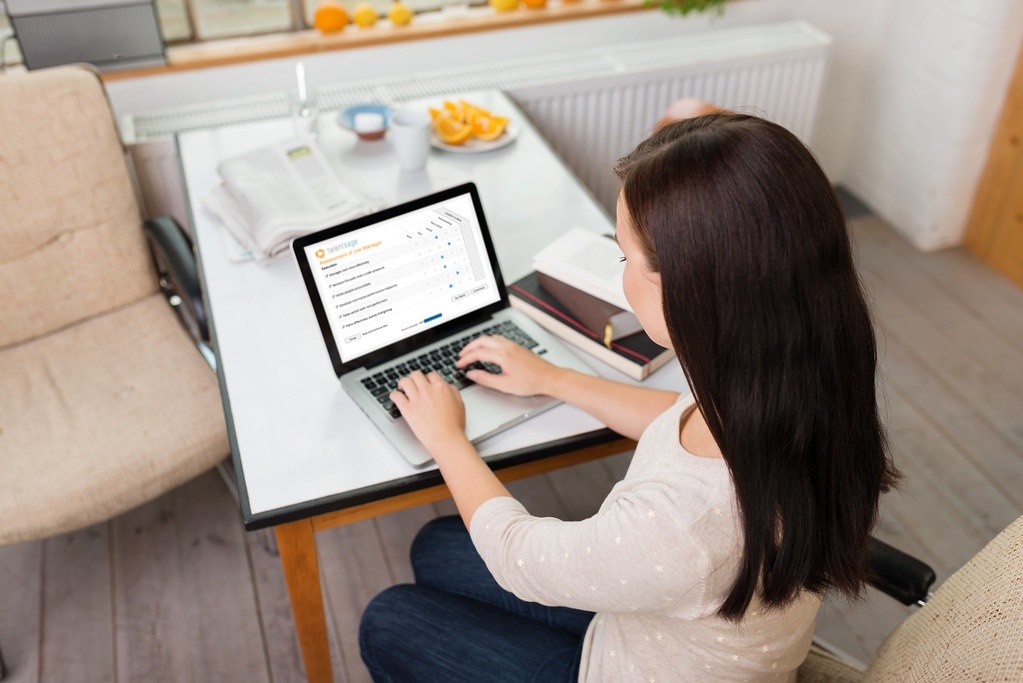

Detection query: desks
(172, 89), (691, 683)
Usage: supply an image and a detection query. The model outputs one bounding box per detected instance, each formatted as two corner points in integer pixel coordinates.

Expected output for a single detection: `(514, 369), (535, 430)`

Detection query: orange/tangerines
(387, 5), (412, 25)
(429, 101), (509, 144)
(354, 3), (377, 26)
(490, 0), (579, 12)
(315, 2), (347, 32)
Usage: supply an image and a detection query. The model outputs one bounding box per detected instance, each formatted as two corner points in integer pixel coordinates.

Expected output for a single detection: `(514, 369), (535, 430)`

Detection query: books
(201, 132), (360, 264)
(507, 227), (677, 382)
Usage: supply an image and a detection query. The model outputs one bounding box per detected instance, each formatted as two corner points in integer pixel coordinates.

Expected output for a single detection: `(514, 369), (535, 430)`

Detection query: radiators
(120, 17), (831, 269)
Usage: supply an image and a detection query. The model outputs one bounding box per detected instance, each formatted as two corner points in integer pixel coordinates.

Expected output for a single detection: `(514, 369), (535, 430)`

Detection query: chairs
(0, 61), (280, 683)
(793, 514), (1023, 683)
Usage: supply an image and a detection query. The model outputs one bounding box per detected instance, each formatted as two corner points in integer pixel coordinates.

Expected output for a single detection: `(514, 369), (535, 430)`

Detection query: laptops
(289, 181), (597, 469)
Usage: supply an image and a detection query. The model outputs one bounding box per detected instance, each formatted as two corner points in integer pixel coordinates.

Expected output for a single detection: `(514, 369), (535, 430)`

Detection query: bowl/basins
(335, 104), (392, 139)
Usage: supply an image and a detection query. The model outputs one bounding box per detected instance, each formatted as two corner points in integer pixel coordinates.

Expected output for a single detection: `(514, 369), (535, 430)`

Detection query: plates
(428, 123), (518, 153)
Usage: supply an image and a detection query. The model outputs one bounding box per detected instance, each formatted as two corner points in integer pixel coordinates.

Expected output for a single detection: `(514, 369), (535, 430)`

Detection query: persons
(357, 110), (906, 683)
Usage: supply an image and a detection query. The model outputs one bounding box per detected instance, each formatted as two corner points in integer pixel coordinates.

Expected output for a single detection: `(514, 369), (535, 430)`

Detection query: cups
(387, 110), (431, 171)
